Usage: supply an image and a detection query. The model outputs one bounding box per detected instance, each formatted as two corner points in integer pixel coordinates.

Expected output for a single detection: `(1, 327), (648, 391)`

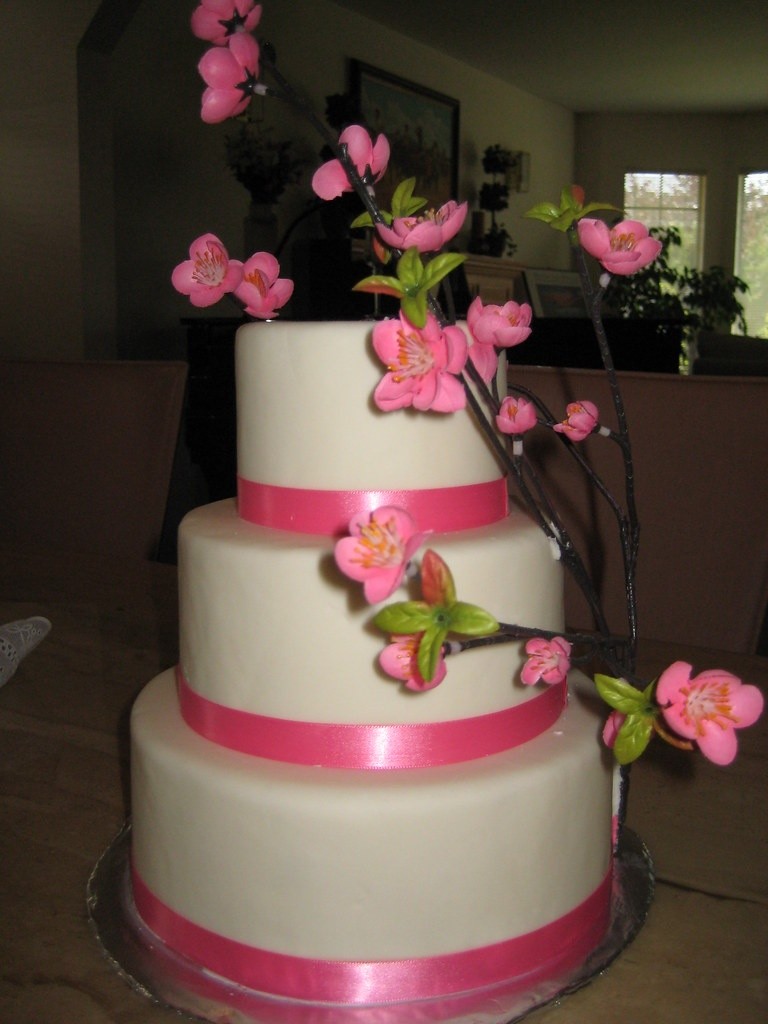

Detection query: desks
(0, 597), (768, 1024)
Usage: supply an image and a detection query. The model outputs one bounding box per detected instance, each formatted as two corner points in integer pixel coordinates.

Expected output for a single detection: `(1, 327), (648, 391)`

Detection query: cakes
(128, 321), (614, 1004)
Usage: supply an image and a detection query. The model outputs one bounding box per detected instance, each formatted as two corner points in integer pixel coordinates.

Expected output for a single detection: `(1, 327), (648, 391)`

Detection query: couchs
(507, 319), (768, 377)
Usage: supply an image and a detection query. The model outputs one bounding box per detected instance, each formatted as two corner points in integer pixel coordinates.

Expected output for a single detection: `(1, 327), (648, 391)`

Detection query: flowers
(172, 0), (766, 767)
(318, 91), (367, 214)
(467, 142), (518, 257)
(221, 121), (305, 204)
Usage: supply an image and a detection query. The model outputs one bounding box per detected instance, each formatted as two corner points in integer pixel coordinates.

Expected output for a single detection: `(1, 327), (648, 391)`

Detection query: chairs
(0, 356), (189, 633)
(506, 365), (768, 655)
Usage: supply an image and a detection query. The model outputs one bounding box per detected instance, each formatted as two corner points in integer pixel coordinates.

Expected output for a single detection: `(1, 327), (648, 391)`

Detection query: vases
(320, 206), (367, 240)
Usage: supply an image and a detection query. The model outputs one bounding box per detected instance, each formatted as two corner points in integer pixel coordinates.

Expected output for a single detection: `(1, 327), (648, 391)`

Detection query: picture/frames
(343, 54), (460, 237)
(522, 266), (592, 318)
